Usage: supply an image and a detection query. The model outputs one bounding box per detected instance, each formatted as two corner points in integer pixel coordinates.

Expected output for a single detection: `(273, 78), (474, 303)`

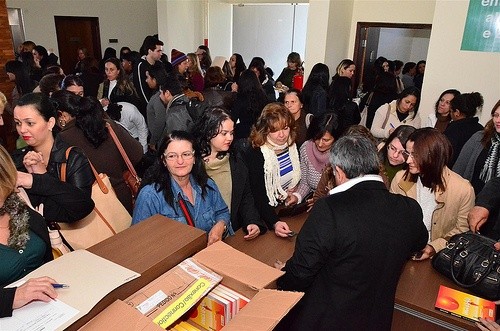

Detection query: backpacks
(169, 96), (213, 130)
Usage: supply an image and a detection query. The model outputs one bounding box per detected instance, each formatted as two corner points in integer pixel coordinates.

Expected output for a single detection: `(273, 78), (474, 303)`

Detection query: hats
(171, 49), (188, 67)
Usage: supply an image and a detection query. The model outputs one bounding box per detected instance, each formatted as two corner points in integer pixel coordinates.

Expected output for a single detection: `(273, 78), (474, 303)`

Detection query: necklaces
(187, 194), (193, 198)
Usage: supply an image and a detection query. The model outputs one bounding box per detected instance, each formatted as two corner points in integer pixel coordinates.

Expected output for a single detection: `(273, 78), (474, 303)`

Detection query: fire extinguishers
(294, 66), (304, 93)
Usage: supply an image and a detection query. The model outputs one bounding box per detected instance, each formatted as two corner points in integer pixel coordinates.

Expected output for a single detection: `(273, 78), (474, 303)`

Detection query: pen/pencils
(51, 284), (70, 288)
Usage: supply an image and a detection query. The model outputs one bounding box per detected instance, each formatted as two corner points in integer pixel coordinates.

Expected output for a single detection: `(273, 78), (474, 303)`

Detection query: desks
(0, 197), (500, 331)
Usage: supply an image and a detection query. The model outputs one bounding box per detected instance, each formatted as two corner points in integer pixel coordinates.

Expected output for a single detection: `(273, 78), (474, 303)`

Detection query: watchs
(224, 223), (228, 232)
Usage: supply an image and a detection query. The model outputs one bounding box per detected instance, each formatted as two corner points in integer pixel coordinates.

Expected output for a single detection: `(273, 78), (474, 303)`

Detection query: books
(436, 286), (495, 325)
(166, 284), (251, 331)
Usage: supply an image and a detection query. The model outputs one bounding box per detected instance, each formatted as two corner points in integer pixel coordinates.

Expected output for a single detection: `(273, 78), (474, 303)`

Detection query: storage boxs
(75, 240), (305, 331)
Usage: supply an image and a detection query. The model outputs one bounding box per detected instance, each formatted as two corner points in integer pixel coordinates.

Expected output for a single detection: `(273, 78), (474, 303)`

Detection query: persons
(369, 87), (500, 261)
(302, 53), (426, 138)
(195, 108), (268, 240)
(45, 88), (145, 226)
(97, 34), (277, 177)
(15, 171), (96, 222)
(13, 91), (99, 198)
(74, 46), (91, 96)
(282, 89), (309, 144)
(251, 102), (313, 239)
(475, 316), (500, 331)
(131, 130), (233, 247)
(0, 41), (65, 151)
(273, 136), (429, 331)
(0, 144), (59, 318)
(272, 51), (304, 91)
(308, 125), (390, 213)
(61, 73), (84, 97)
(282, 113), (339, 206)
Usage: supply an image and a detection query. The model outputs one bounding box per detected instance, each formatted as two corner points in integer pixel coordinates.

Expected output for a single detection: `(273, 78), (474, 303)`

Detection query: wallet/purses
(275, 192), (313, 217)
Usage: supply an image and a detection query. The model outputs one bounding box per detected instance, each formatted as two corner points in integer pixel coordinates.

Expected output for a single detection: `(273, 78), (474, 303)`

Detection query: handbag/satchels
(430, 231), (500, 301)
(358, 92), (373, 128)
(106, 121), (142, 203)
(58, 146), (132, 250)
(376, 103), (391, 146)
(17, 187), (71, 259)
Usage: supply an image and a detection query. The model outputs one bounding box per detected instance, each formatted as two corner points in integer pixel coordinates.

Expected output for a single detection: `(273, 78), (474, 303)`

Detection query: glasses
(163, 150), (196, 161)
(387, 144), (405, 156)
(403, 152), (414, 159)
(491, 112), (500, 117)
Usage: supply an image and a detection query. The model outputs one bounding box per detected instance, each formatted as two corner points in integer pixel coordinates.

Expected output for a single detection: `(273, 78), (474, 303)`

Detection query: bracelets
(274, 221), (280, 227)
